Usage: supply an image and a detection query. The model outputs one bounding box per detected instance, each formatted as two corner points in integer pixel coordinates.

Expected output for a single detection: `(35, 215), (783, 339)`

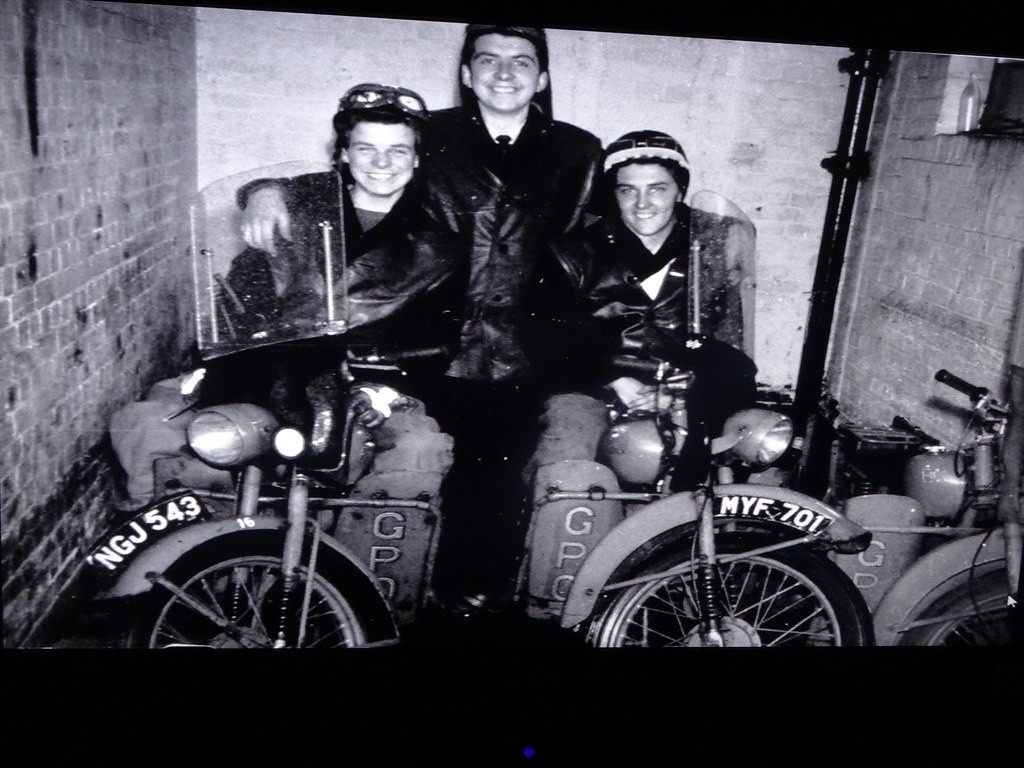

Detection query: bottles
(957, 72), (981, 133)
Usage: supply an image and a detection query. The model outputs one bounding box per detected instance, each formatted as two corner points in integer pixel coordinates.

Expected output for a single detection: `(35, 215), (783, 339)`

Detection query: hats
(604, 130), (691, 174)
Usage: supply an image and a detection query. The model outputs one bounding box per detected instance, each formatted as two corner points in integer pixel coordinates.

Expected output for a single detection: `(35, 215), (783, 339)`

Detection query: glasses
(338, 82), (429, 122)
(608, 136), (679, 152)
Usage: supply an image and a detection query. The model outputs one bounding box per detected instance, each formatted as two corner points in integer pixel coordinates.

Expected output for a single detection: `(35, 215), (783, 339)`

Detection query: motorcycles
(86, 159), (442, 651)
(794, 369), (1024, 646)
(510, 191), (875, 647)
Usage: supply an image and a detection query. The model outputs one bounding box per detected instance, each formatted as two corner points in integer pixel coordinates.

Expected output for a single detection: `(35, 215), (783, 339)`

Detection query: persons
(533, 129), (757, 493)
(85, 82), (468, 575)
(236, 22), (607, 615)
